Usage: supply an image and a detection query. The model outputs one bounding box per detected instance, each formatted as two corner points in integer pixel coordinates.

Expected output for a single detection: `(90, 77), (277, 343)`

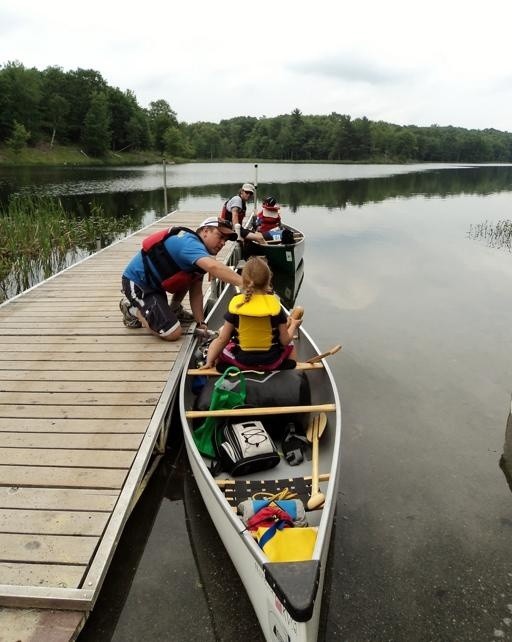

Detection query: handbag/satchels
(194, 390), (246, 454)
(221, 417), (280, 478)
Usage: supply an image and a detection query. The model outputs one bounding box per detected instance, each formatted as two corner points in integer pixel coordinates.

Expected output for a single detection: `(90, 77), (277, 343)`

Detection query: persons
(220, 183), (268, 246)
(253, 195), (283, 234)
(118, 216), (244, 342)
(197, 256), (304, 370)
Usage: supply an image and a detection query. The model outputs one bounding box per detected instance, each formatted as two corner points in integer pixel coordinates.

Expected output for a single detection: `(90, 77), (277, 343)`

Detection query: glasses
(205, 221), (233, 229)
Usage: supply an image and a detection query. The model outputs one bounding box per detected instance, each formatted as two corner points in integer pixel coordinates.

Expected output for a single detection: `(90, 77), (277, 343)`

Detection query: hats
(262, 195), (277, 207)
(195, 217), (238, 241)
(243, 183), (255, 193)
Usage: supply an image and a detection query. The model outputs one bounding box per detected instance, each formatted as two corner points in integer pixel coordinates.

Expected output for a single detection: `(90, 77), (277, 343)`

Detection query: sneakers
(170, 303), (195, 323)
(119, 298), (142, 328)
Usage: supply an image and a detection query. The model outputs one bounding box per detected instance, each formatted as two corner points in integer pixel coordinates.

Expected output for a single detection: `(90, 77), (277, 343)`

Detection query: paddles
(306, 412), (327, 509)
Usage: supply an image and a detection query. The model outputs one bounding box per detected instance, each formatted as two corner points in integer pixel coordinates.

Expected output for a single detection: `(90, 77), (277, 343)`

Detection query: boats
(270, 257), (305, 310)
(246, 222), (306, 274)
(177, 258), (345, 642)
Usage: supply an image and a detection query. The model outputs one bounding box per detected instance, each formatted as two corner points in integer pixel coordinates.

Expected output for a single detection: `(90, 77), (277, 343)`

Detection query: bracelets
(195, 320), (210, 327)
